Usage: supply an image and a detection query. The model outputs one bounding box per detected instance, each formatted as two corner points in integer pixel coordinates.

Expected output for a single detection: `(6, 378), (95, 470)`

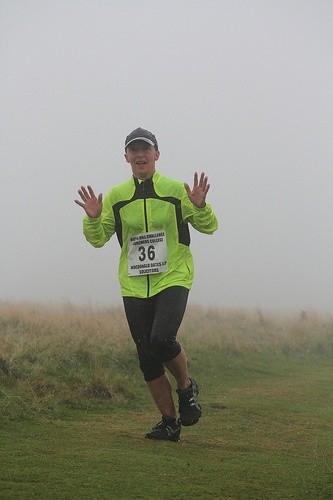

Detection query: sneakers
(145, 415), (182, 442)
(176, 377), (202, 426)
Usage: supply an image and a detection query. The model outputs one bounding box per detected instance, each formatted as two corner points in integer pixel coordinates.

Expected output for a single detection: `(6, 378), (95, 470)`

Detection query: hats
(125, 127), (158, 154)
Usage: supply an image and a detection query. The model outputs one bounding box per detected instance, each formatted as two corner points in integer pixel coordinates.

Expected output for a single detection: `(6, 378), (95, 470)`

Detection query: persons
(74, 126), (218, 441)
